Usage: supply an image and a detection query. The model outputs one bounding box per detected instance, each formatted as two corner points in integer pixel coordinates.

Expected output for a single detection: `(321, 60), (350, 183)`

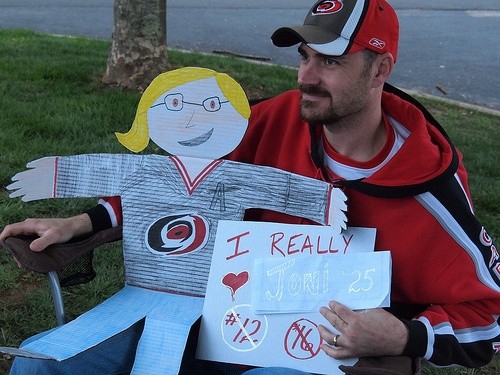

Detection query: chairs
(3, 224), (422, 375)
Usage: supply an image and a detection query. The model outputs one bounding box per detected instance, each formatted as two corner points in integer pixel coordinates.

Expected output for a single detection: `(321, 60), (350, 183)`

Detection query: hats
(271, 0), (400, 64)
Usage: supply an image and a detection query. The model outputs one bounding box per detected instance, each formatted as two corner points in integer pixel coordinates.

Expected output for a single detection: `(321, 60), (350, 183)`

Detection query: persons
(0, 0), (500, 375)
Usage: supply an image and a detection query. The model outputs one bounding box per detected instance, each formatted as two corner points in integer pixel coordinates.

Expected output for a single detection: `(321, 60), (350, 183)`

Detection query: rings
(333, 335), (340, 347)
